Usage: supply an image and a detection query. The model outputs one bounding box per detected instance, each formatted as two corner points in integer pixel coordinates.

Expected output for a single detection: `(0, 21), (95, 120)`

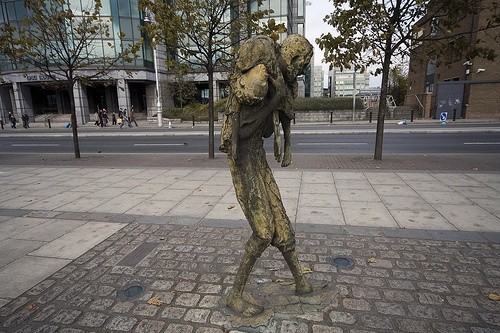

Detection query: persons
(93, 108), (108, 127)
(8, 112), (30, 130)
(219, 33), (313, 318)
(120, 109), (132, 129)
(112, 111), (117, 126)
(218, 35), (297, 168)
(128, 109), (138, 128)
(117, 108), (124, 126)
